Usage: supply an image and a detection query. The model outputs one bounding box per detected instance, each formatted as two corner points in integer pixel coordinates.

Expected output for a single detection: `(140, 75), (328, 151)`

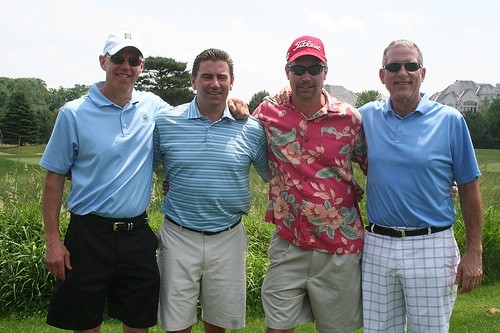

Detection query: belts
(365, 223), (452, 237)
(165, 214), (242, 235)
(79, 215), (150, 233)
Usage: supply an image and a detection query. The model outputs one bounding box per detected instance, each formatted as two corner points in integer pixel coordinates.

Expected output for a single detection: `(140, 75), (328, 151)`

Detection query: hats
(103, 21), (146, 60)
(286, 36), (327, 64)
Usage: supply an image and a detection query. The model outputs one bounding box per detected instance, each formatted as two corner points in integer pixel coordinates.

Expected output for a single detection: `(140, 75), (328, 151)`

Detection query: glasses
(288, 64), (325, 76)
(105, 54), (144, 67)
(383, 62), (422, 72)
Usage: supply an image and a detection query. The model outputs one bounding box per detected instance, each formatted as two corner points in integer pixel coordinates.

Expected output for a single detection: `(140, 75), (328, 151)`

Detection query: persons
(274, 40), (483, 333)
(64, 48), (365, 333)
(39, 30), (250, 333)
(162, 36), (458, 333)
